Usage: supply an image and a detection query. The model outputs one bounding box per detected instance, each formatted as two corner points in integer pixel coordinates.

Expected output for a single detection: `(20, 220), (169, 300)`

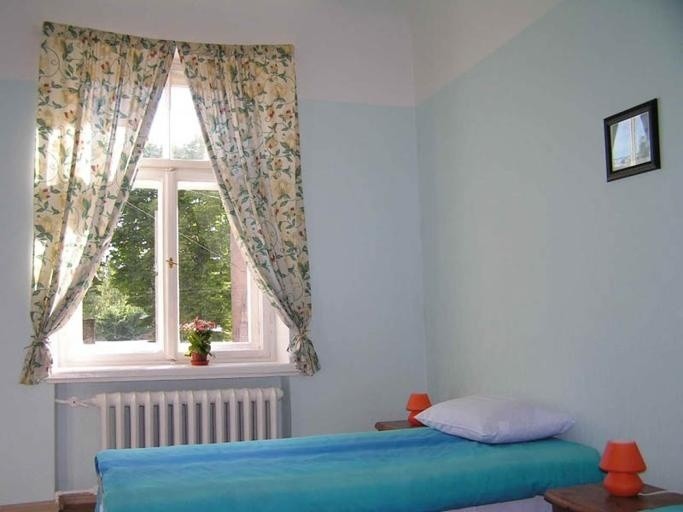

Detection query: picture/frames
(603, 97), (660, 182)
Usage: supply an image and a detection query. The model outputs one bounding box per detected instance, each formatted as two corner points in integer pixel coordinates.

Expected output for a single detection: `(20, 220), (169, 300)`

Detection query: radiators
(91, 386), (286, 449)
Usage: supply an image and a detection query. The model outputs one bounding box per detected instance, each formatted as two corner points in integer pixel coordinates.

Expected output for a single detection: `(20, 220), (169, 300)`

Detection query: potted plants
(184, 328), (212, 365)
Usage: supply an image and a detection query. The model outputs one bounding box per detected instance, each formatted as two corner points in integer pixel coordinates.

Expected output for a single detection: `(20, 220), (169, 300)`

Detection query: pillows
(413, 395), (580, 443)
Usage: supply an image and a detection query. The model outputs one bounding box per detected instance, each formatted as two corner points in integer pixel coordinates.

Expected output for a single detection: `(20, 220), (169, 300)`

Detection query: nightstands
(375, 419), (426, 430)
(543, 478), (683, 512)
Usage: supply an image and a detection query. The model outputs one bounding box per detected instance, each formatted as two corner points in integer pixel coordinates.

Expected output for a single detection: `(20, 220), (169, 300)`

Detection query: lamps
(406, 393), (432, 427)
(598, 440), (646, 497)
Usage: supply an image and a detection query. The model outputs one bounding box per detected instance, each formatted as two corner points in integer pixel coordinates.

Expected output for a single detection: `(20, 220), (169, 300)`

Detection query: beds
(93, 426), (605, 512)
